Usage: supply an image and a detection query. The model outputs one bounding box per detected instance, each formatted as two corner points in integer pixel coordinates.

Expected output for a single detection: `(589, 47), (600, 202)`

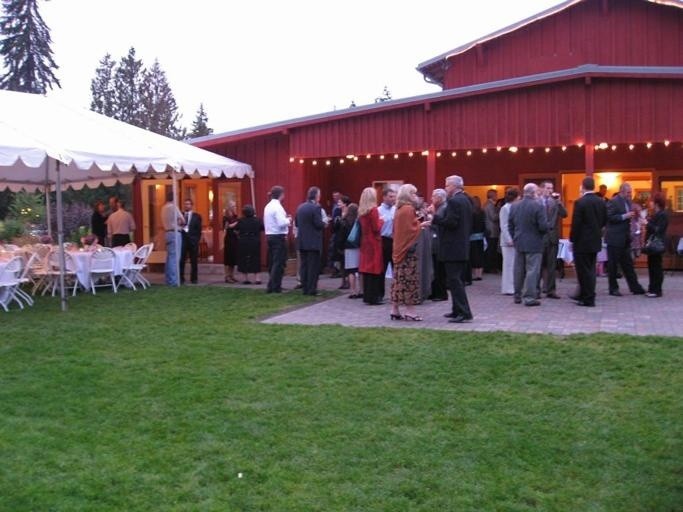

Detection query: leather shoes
(443, 312), (472, 322)
(515, 292), (561, 306)
(330, 273), (386, 305)
(568, 295), (595, 307)
(609, 290), (662, 297)
(426, 294), (447, 301)
(267, 283), (323, 297)
(225, 276), (260, 284)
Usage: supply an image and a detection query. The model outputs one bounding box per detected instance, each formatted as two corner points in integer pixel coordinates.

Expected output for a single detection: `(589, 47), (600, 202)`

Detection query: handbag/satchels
(347, 219), (361, 246)
(644, 239), (666, 255)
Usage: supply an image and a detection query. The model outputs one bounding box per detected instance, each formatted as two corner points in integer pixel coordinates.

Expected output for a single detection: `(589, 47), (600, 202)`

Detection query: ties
(545, 199), (548, 214)
(185, 213), (188, 225)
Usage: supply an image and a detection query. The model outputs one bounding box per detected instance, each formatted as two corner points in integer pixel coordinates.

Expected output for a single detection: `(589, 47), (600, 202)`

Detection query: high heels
(391, 313), (423, 321)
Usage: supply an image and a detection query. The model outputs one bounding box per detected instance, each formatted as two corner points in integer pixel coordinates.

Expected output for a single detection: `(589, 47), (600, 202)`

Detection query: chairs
(0, 238), (155, 313)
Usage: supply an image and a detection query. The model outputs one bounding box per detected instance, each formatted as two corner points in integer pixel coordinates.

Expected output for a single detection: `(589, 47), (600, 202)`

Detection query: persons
(179, 198), (202, 286)
(91, 196), (137, 248)
(160, 191), (187, 287)
(333, 176), (450, 304)
(389, 184), (431, 320)
(424, 176), (474, 323)
(223, 186), (328, 296)
(472, 178), (667, 307)
